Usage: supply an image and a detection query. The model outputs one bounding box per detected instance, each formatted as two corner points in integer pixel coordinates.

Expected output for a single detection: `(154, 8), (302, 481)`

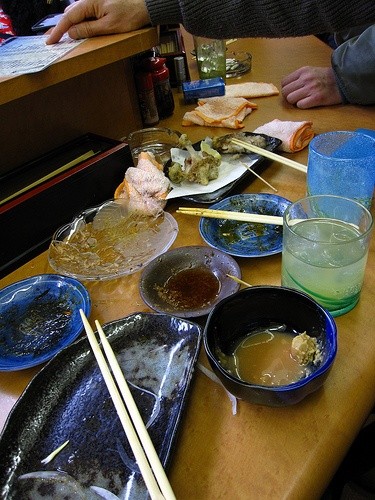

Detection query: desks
(0, 24), (375, 500)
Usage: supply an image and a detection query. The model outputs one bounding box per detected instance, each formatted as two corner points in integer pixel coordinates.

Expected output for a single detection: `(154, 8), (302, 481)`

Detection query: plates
(139, 247), (242, 317)
(0, 274), (91, 371)
(48, 198), (180, 282)
(0, 313), (202, 499)
(201, 193), (309, 259)
(162, 132), (283, 204)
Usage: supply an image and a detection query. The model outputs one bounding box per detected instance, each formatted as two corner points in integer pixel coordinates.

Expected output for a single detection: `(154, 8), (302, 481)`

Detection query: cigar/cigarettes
(226, 63), (239, 71)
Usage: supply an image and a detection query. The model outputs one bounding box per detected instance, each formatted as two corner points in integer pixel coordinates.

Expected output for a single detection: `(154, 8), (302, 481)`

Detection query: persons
(46, 0), (375, 109)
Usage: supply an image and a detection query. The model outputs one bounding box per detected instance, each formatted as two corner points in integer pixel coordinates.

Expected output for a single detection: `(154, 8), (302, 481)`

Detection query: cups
(282, 195), (372, 316)
(306, 131), (375, 227)
(193, 34), (227, 87)
(126, 127), (183, 165)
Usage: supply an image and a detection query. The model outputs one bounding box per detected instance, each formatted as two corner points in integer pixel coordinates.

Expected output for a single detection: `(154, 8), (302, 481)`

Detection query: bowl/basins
(205, 286), (336, 407)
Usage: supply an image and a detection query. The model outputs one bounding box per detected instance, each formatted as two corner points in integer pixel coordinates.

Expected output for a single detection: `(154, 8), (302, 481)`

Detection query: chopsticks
(78, 308), (178, 500)
(191, 39), (236, 60)
(177, 207), (307, 227)
(230, 138), (307, 176)
